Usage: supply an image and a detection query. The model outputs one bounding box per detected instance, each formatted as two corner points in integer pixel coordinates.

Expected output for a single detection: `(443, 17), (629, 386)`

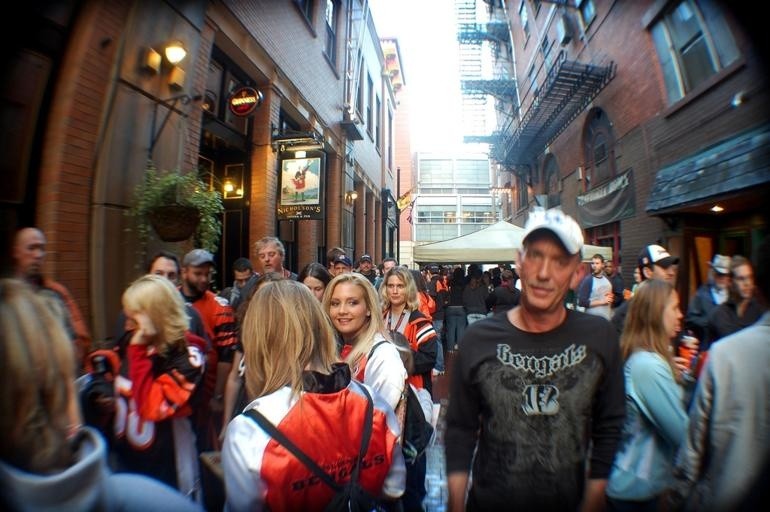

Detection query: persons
(441, 209), (625, 512)
(579, 246), (769, 512)
(0, 230), (521, 512)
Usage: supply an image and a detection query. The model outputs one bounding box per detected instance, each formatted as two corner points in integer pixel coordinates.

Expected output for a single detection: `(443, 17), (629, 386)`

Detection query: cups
(680, 336), (700, 369)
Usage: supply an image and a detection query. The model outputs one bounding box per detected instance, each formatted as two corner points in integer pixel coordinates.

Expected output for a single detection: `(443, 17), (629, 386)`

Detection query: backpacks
(368, 341), (441, 466)
(242, 378), (390, 512)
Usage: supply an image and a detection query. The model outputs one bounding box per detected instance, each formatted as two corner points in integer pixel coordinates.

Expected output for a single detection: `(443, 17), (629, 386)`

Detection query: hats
(360, 255), (371, 262)
(333, 255), (352, 266)
(705, 255), (733, 275)
(182, 248), (213, 267)
(638, 244), (680, 268)
(522, 208), (584, 261)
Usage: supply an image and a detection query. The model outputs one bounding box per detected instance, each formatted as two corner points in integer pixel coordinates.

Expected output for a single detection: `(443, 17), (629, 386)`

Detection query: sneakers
(432, 368), (445, 376)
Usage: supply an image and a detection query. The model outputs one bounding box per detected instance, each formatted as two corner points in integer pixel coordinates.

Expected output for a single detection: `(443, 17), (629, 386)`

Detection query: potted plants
(121, 174), (222, 274)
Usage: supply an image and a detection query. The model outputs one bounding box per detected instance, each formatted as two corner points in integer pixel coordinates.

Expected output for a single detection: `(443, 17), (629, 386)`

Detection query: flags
(395, 193), (411, 210)
(406, 202), (417, 223)
(386, 49), (401, 95)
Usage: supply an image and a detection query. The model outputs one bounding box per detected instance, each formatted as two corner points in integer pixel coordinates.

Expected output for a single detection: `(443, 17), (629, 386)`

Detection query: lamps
(221, 175), (236, 192)
(164, 36), (188, 64)
(349, 189), (360, 202)
